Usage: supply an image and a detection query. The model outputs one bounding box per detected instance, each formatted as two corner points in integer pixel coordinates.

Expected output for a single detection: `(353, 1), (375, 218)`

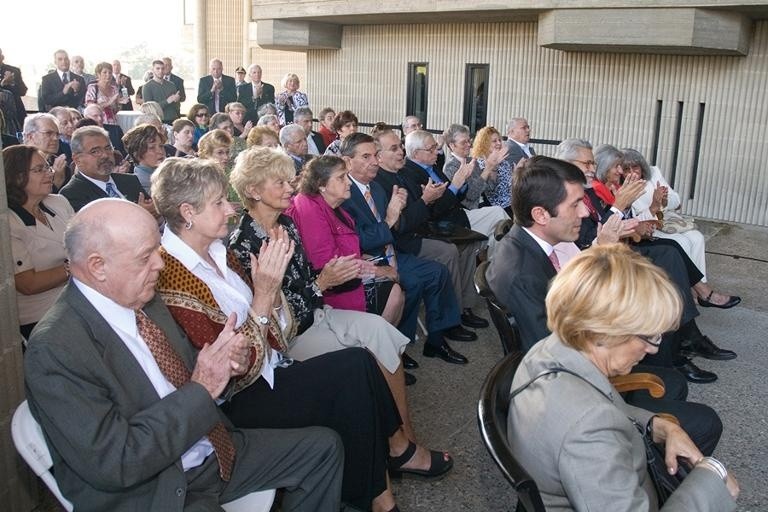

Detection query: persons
(0, 49), (308, 126)
(486, 137), (741, 512)
(0, 107), (536, 512)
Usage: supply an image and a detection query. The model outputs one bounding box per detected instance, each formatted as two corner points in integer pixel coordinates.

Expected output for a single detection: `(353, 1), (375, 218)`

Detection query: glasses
(637, 334), (663, 348)
(194, 112), (209, 118)
(569, 159), (597, 169)
(76, 143), (116, 158)
(25, 164), (55, 176)
(35, 130), (62, 139)
(415, 141), (438, 154)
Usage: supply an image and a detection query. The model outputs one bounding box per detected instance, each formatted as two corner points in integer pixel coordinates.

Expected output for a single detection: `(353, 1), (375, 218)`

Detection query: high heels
(384, 438), (454, 484)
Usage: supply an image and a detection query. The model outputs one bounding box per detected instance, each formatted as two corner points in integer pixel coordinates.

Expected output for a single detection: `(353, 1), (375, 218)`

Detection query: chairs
(10, 397), (276, 511)
(477, 350), (680, 511)
(470, 257), (667, 399)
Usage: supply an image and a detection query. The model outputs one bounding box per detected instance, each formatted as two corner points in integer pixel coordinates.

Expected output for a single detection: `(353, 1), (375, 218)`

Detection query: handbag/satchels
(642, 434), (691, 508)
(77, 104), (85, 115)
(413, 219), (488, 244)
(656, 210), (696, 234)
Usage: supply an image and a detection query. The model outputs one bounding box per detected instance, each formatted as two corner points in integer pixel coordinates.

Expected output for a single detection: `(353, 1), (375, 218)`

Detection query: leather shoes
(442, 325), (478, 342)
(697, 290), (741, 309)
(403, 371), (416, 386)
(423, 338), (469, 364)
(678, 335), (737, 360)
(401, 351), (419, 369)
(461, 308), (488, 327)
(672, 358), (717, 383)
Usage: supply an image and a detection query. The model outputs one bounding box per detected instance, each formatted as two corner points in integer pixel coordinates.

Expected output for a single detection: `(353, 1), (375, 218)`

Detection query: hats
(235, 66), (246, 74)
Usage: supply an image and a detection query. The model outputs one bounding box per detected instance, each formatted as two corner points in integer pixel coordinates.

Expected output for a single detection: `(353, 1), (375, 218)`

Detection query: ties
(365, 188), (398, 271)
(105, 183), (120, 198)
(550, 250), (561, 275)
(62, 73), (68, 84)
(135, 309), (236, 483)
